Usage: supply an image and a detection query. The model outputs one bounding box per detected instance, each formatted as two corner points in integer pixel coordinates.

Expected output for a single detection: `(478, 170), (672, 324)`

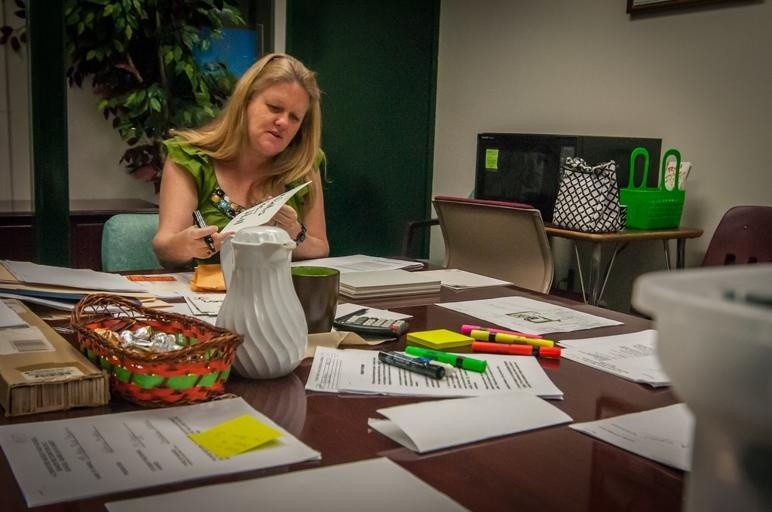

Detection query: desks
(543, 221), (704, 307)
(0, 254), (697, 512)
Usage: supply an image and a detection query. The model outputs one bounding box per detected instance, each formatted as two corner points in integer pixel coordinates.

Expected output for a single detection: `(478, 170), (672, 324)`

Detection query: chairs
(101, 212), (195, 274)
(699, 205), (772, 277)
(400, 195), (555, 298)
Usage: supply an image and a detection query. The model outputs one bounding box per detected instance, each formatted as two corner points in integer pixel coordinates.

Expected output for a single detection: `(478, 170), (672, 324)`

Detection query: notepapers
(407, 329), (475, 351)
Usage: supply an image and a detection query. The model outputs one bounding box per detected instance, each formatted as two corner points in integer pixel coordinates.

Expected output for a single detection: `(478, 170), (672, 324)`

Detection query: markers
(192, 210), (215, 253)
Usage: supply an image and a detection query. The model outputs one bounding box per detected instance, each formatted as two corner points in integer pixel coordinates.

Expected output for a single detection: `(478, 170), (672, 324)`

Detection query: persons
(151, 53), (331, 273)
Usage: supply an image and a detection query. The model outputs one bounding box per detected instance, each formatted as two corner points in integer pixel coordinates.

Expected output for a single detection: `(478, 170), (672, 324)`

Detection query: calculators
(333, 309), (409, 338)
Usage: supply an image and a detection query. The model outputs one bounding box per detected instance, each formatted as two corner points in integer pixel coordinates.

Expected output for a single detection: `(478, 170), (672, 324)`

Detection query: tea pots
(214, 225), (308, 380)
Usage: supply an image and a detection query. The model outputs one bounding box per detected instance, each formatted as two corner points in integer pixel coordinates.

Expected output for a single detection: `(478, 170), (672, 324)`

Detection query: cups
(291, 264), (339, 333)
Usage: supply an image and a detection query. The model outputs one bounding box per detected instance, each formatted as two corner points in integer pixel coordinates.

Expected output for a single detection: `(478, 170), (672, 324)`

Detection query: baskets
(68, 291), (246, 411)
(619, 146), (687, 231)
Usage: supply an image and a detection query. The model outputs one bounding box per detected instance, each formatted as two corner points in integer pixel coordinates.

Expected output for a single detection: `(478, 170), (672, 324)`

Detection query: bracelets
(294, 224), (307, 250)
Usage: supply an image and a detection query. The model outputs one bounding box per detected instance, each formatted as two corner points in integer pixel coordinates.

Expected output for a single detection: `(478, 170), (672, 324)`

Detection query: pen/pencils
(378, 324), (567, 379)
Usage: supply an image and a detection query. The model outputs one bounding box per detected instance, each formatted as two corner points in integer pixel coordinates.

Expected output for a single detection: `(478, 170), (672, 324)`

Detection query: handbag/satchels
(552, 157), (629, 234)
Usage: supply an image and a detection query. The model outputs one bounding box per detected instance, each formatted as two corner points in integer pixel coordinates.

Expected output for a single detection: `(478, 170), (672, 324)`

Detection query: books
(0, 259), (174, 311)
(339, 268), (442, 299)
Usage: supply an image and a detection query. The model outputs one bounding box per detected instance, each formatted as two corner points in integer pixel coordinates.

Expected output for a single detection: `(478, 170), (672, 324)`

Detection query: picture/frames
(625, 0), (764, 14)
